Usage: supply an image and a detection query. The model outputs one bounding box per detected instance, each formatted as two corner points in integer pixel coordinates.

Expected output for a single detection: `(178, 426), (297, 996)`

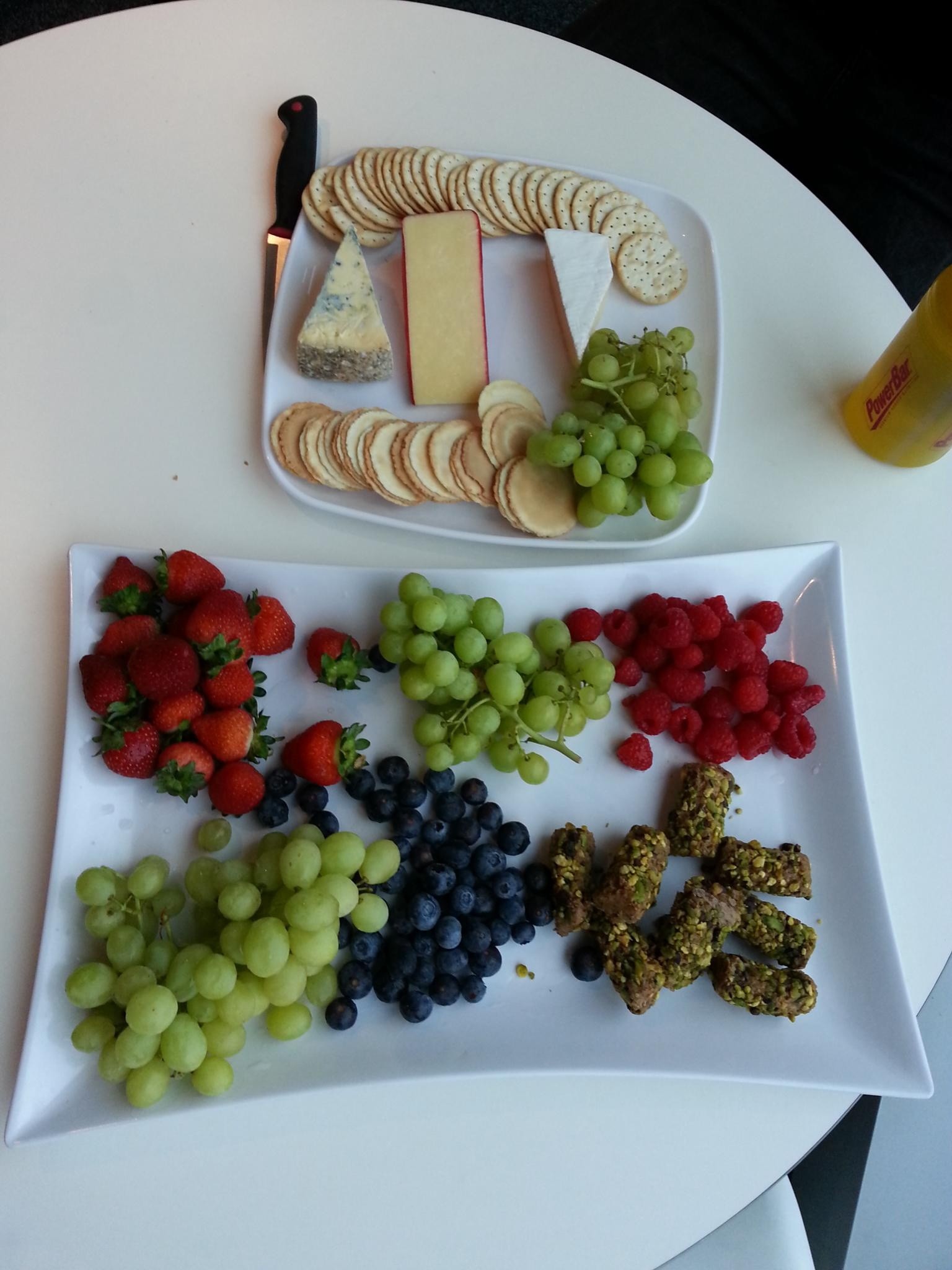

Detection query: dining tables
(1, 0), (952, 1269)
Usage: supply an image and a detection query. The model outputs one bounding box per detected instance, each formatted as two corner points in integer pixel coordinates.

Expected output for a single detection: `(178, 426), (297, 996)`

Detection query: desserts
(293, 227), (395, 384)
(541, 229), (615, 371)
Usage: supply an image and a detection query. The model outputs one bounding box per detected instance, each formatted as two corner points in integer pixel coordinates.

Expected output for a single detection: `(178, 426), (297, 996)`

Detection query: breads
(400, 209), (491, 406)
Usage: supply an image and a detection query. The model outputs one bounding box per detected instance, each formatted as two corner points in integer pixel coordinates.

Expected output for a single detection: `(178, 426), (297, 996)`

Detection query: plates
(0, 534), (932, 1162)
(265, 145), (723, 547)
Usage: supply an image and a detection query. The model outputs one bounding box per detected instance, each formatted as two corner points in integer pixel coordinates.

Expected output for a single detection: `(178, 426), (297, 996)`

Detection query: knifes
(258, 92), (321, 382)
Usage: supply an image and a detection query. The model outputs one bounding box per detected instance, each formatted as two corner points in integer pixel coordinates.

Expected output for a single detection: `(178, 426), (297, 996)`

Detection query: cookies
(269, 380), (578, 538)
(300, 145), (690, 304)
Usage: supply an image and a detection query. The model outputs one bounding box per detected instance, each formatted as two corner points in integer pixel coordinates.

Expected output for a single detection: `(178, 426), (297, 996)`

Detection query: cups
(845, 224), (952, 469)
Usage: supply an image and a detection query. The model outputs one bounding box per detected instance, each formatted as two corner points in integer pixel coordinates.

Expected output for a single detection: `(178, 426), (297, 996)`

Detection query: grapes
(375, 571), (615, 785)
(63, 817), (401, 1109)
(525, 326), (714, 528)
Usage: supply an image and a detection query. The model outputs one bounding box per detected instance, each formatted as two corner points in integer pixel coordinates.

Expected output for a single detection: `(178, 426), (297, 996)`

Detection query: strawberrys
(80, 548), (372, 820)
